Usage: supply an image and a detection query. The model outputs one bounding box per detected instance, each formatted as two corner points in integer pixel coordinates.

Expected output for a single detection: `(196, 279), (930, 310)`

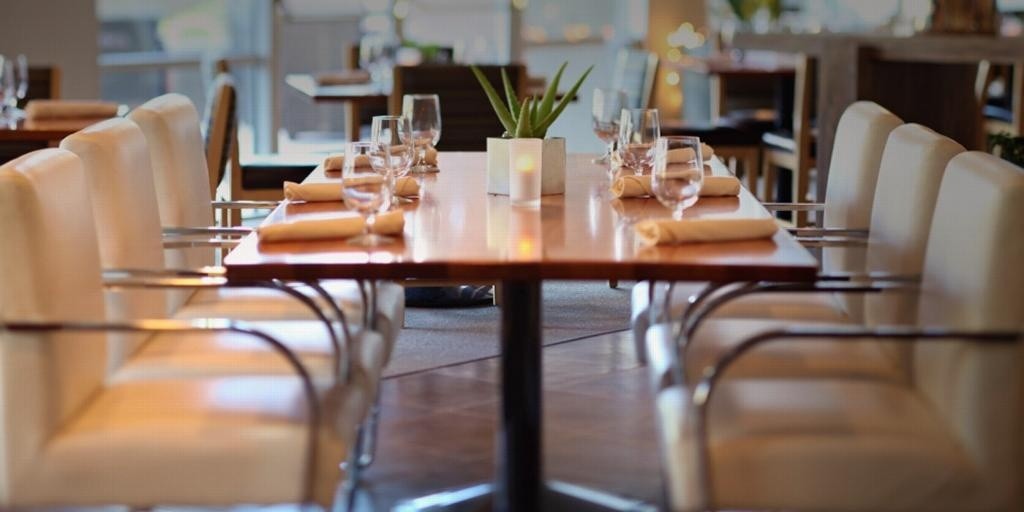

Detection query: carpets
(379, 280), (636, 383)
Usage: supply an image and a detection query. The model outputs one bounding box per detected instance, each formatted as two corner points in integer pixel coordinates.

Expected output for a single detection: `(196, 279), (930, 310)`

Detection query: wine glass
(0, 52), (32, 132)
(590, 88), (705, 223)
(340, 92), (442, 246)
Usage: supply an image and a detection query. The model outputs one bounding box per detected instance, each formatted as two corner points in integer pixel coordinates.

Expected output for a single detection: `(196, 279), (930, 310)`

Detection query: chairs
(4, 146), (368, 509)
(2, 62), (240, 199)
(655, 149), (1022, 509)
(55, 113), (390, 510)
(127, 92), (405, 471)
(628, 98), (904, 367)
(608, 46), (1021, 226)
(643, 122), (967, 509)
(345, 40), (530, 149)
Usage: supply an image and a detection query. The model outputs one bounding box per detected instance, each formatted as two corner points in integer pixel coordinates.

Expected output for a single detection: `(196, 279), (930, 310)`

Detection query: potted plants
(470, 63), (598, 198)
